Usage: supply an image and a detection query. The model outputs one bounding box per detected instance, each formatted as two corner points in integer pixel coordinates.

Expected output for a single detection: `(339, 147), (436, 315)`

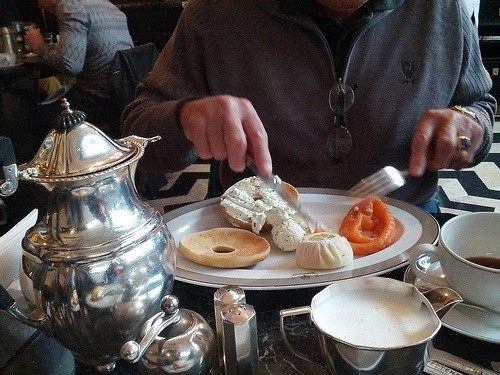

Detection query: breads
(223, 177), (300, 231)
(179, 226), (270, 268)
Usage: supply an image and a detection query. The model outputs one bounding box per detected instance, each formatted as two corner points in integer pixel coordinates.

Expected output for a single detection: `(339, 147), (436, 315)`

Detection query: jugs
(0, 98), (176, 375)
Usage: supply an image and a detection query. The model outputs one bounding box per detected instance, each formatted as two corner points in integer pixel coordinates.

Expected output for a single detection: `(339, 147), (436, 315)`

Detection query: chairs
(108, 42), (158, 102)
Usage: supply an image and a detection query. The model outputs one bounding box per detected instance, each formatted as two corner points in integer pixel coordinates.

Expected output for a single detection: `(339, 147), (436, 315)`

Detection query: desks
(0, 61), (63, 161)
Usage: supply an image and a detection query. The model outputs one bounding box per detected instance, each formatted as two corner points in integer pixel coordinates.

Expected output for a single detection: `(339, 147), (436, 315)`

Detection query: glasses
(327, 78), (356, 159)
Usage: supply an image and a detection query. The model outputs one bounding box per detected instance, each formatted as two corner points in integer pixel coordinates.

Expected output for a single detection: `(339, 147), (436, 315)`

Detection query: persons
(120, 0), (497, 212)
(25, 0), (135, 125)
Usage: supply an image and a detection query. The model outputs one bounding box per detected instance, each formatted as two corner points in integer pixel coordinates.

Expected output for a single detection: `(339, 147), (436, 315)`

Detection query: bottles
(214, 285), (260, 375)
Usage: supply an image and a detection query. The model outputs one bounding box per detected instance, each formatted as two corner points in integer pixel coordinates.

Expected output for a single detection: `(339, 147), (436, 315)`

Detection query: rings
(457, 136), (471, 151)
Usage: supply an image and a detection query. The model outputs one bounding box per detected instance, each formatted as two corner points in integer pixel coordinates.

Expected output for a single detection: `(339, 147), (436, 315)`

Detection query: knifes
(244, 154), (322, 226)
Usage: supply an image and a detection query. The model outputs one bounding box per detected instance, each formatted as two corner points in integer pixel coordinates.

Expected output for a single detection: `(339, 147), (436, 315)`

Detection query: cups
(279, 278), (464, 375)
(41, 32), (52, 45)
(6, 55), (20, 64)
(12, 22), (32, 54)
(409, 212), (500, 312)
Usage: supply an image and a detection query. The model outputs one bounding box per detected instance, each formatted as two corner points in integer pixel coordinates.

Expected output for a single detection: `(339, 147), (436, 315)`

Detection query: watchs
(452, 104), (476, 119)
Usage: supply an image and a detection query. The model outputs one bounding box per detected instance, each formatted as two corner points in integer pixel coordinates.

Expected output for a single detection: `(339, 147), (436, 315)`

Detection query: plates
(162, 187), (441, 290)
(0, 53), (24, 67)
(403, 254), (500, 343)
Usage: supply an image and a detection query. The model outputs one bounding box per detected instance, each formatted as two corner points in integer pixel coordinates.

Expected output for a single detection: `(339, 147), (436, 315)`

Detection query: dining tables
(0, 213), (500, 375)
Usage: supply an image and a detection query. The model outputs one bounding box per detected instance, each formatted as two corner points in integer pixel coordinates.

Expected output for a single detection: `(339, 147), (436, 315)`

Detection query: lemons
(301, 232), (340, 242)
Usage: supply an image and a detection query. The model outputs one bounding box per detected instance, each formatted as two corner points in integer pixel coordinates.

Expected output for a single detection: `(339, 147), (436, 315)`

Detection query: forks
(346, 156), (435, 200)
